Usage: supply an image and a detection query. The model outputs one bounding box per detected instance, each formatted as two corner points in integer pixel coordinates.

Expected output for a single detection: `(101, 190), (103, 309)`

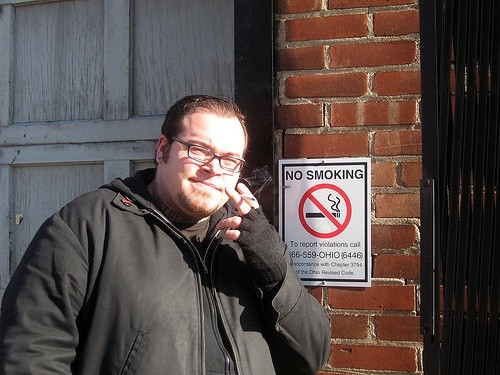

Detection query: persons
(0, 93), (332, 374)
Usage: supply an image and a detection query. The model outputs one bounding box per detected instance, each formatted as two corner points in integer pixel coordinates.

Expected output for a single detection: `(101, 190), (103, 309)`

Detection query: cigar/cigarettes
(239, 193), (258, 202)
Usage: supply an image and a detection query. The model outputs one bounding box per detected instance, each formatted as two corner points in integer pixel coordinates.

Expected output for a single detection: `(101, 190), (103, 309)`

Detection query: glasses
(172, 135), (247, 173)
